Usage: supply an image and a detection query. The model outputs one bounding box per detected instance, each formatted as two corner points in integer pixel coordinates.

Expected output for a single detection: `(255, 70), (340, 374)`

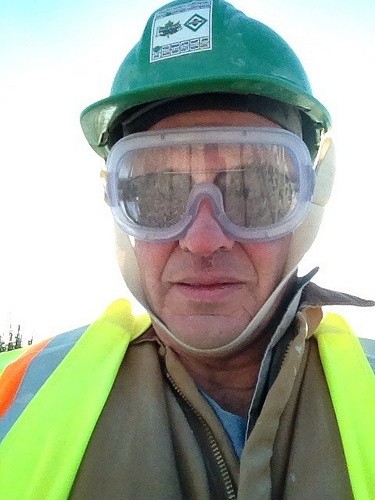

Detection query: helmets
(80, 0), (333, 160)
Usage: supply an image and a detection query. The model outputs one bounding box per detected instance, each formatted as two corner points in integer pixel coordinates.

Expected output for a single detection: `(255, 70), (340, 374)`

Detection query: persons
(1, 0), (375, 500)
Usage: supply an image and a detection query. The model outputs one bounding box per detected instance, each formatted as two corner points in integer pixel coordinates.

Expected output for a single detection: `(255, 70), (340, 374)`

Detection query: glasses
(104, 127), (316, 243)
